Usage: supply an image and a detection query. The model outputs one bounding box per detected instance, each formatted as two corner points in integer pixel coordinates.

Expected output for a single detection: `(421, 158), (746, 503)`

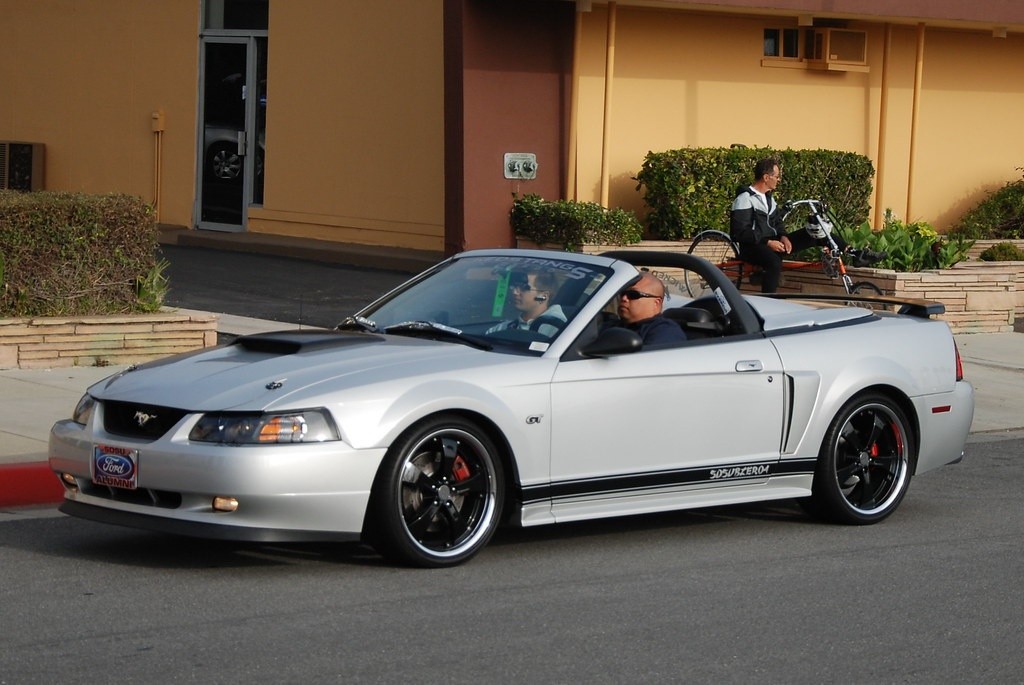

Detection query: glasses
(510, 280), (547, 293)
(769, 174), (779, 179)
(620, 288), (663, 300)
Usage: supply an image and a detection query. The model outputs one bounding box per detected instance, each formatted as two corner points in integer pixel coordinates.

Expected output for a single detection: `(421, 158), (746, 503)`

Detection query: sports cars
(45, 248), (977, 568)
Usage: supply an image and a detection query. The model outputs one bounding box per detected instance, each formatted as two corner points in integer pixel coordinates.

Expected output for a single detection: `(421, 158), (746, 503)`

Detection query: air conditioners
(814, 27), (868, 66)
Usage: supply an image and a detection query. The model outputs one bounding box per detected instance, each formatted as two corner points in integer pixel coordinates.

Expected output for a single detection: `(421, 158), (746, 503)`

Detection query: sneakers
(852, 248), (887, 267)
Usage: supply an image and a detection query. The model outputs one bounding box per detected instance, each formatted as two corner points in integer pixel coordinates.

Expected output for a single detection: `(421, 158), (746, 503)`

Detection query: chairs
(663, 304), (732, 338)
(558, 291), (605, 348)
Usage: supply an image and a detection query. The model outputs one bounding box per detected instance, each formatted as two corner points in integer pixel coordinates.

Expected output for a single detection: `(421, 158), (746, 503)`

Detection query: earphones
(534, 296), (545, 302)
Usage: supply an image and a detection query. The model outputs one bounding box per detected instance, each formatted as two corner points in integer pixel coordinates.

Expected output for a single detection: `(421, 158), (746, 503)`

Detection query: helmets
(804, 212), (833, 239)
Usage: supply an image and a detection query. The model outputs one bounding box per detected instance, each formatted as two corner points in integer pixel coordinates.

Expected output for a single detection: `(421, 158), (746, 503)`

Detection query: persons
(598, 269), (687, 358)
(730, 158), (887, 296)
(485, 265), (569, 344)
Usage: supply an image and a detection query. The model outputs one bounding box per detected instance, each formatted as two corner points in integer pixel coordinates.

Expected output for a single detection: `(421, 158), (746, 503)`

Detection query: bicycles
(685, 195), (890, 314)
